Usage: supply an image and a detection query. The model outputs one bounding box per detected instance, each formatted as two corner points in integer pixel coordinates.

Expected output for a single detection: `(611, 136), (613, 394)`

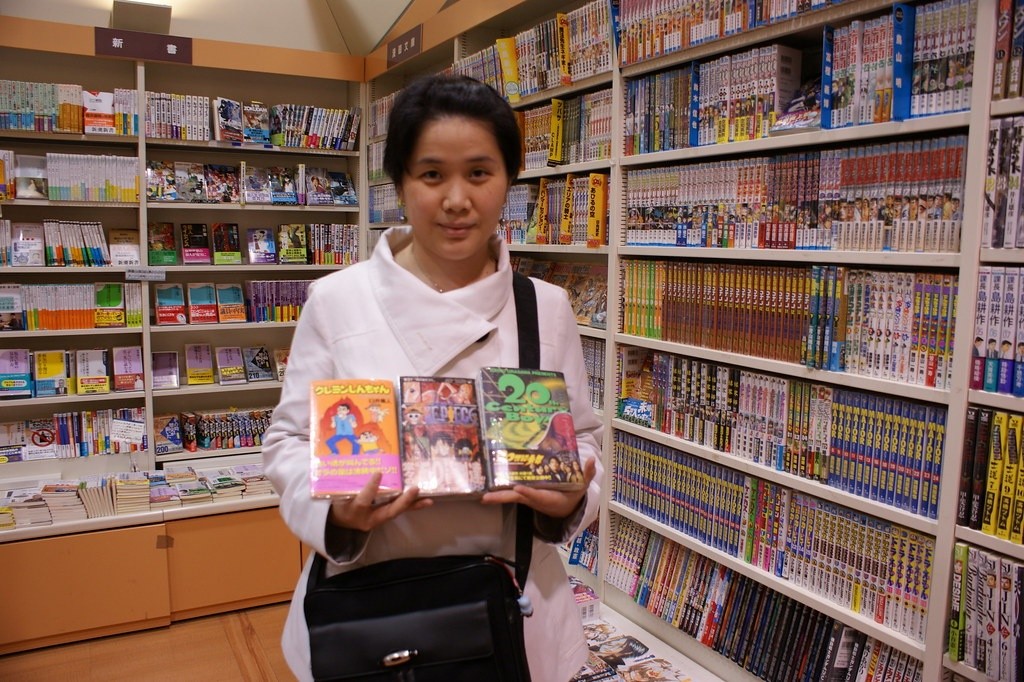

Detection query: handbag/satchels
(303, 553), (533, 682)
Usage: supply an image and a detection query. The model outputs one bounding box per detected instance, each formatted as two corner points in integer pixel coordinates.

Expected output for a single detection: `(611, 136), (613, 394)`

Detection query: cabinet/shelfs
(0, 15), (363, 657)
(363, 0), (1024, 682)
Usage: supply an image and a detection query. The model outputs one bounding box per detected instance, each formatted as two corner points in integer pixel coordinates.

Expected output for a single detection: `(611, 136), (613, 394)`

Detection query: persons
(262, 73), (604, 682)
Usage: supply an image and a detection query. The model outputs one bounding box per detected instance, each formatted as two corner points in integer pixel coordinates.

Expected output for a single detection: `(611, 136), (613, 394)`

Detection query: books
(476, 366), (587, 490)
(0, 81), (148, 462)
(0, 464), (272, 533)
(367, 68), (450, 259)
(145, 92), (364, 456)
(603, 0), (1024, 682)
(309, 366), (404, 502)
(397, 376), (486, 502)
(513, 576), (693, 682)
(452, 0), (612, 575)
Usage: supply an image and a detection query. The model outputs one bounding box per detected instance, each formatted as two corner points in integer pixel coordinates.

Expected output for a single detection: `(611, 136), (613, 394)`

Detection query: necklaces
(411, 242), (449, 293)
